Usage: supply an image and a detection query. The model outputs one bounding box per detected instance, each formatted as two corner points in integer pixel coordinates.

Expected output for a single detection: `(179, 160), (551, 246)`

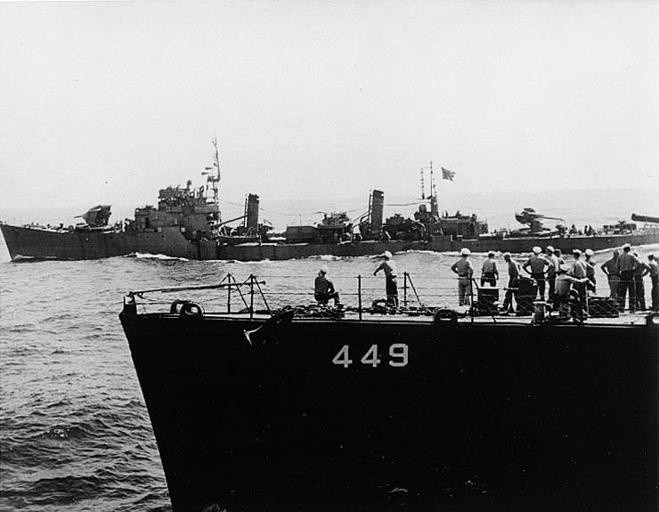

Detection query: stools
(317, 300), (328, 308)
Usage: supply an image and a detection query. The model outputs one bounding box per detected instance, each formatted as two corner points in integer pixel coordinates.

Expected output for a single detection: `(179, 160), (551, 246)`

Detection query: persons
(451, 246), (659, 313)
(314, 269), (339, 306)
(373, 250), (399, 306)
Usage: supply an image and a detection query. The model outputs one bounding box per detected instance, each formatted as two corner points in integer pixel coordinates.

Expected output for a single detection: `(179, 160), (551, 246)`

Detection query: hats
(384, 250), (393, 259)
(460, 247), (471, 255)
(622, 242), (631, 247)
(559, 263), (570, 271)
(532, 245), (593, 256)
(321, 266), (329, 274)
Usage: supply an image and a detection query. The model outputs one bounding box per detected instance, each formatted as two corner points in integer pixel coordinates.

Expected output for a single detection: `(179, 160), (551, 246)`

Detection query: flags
(441, 167), (455, 182)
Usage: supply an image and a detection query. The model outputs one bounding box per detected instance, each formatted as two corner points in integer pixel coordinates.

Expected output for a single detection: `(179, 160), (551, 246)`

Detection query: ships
(117, 270), (659, 512)
